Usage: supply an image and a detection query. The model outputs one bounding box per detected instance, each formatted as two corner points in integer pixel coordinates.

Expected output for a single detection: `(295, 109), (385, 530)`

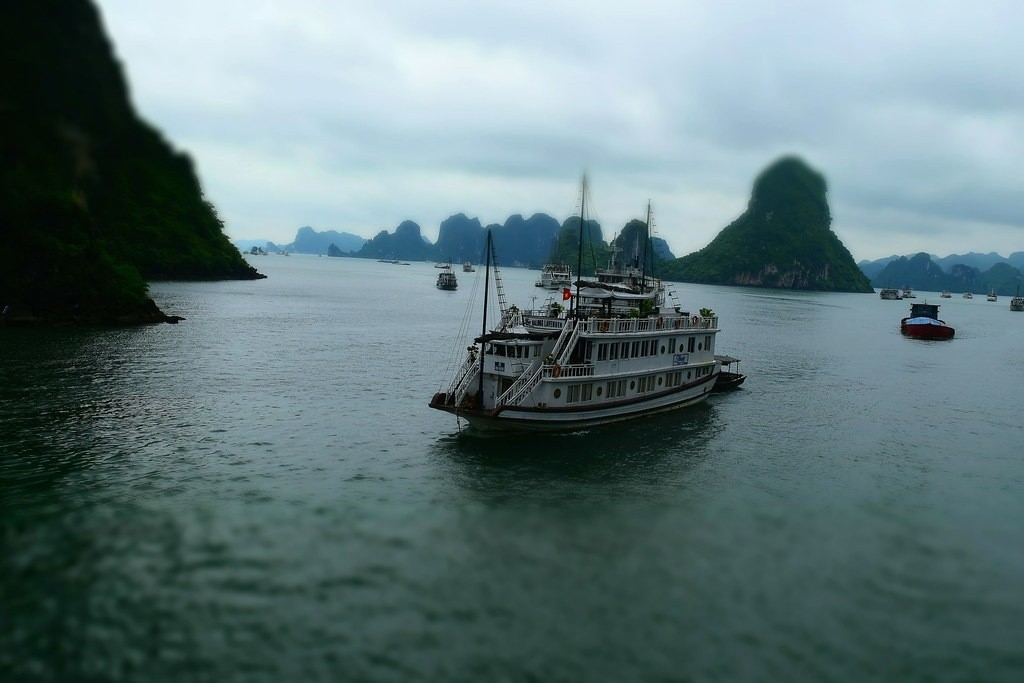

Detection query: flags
(563, 288), (571, 300)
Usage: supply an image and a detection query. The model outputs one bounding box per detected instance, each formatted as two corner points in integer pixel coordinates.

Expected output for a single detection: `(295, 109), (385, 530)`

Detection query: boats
(463, 261), (475, 272)
(902, 288), (917, 298)
(880, 289), (903, 300)
(714, 354), (747, 391)
(986, 293), (997, 301)
(1010, 297), (1024, 311)
(541, 262), (573, 290)
(434, 257), (452, 269)
(963, 293), (973, 299)
(535, 280), (543, 287)
(429, 177), (723, 432)
(900, 303), (955, 338)
(941, 291), (952, 298)
(436, 272), (458, 290)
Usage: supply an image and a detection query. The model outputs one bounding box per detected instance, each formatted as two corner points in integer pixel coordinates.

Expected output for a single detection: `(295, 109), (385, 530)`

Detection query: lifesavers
(657, 316), (663, 326)
(554, 364), (561, 377)
(600, 321), (608, 332)
(693, 315), (697, 325)
